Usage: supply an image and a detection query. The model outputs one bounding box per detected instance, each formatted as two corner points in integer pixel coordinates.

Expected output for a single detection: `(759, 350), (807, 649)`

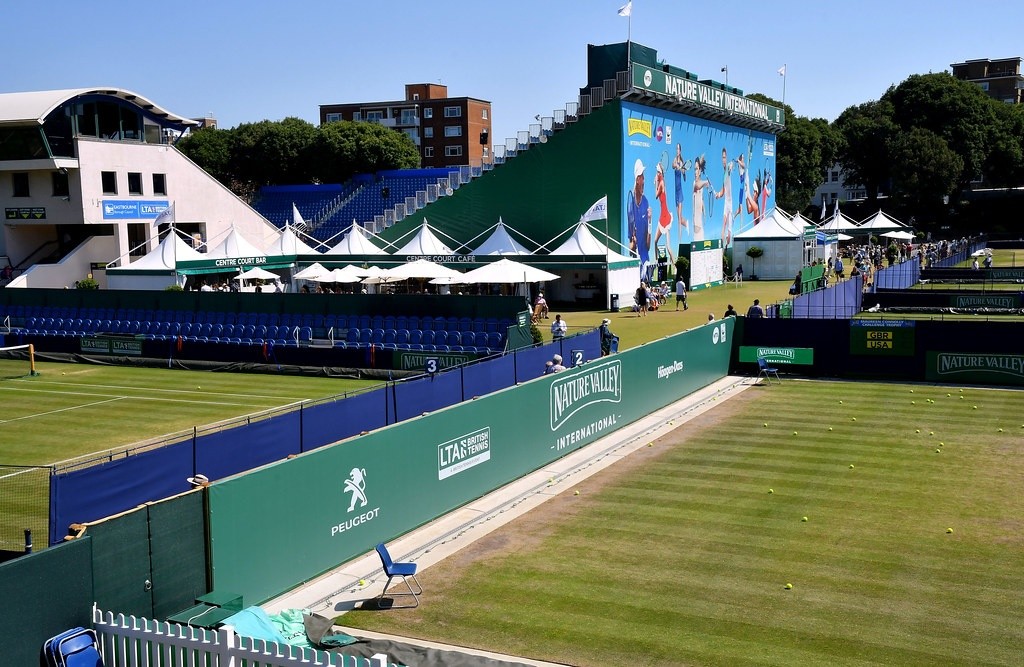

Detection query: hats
(661, 281), (666, 285)
(634, 159), (645, 177)
(867, 283), (871, 285)
(656, 164), (664, 174)
(539, 293), (544, 297)
(988, 253), (993, 257)
(601, 318), (611, 325)
(752, 180), (759, 192)
(835, 257), (840, 261)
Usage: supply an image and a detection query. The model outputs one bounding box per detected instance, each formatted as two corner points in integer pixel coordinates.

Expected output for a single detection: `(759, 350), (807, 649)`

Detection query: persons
(188, 474), (209, 488)
(793, 237), (992, 293)
(198, 280), (550, 319)
(543, 314), (615, 374)
(927, 232), (932, 241)
(628, 145), (773, 282)
(724, 264), (743, 282)
(709, 300), (763, 323)
(633, 277), (688, 317)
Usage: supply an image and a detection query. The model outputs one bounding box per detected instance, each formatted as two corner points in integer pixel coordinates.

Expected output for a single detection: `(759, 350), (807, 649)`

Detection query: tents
(732, 209), (912, 279)
(107, 225), (640, 310)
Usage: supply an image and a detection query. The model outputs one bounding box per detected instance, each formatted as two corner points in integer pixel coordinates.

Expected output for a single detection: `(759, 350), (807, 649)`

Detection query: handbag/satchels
(840, 273), (845, 278)
(789, 284), (796, 294)
(983, 258), (992, 267)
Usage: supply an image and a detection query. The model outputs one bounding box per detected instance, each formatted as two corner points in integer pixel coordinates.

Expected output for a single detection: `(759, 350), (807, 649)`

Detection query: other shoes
(545, 316), (549, 319)
(638, 314), (641, 317)
(686, 221), (690, 236)
(684, 307), (689, 310)
(676, 309), (680, 311)
(644, 313), (646, 316)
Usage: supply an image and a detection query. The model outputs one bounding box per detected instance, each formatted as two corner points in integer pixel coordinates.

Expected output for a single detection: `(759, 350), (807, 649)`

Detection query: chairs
(376, 541), (422, 608)
(755, 359), (782, 387)
(0, 305), (516, 355)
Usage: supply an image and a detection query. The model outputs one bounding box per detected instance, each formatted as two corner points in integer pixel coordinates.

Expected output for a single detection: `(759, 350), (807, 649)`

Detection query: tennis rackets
(627, 190), (637, 258)
(709, 191), (714, 217)
(659, 150), (669, 173)
(765, 158), (771, 177)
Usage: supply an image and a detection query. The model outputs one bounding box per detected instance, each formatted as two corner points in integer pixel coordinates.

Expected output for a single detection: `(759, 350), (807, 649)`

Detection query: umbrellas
(880, 231), (916, 244)
(234, 258), (561, 302)
(835, 233), (852, 240)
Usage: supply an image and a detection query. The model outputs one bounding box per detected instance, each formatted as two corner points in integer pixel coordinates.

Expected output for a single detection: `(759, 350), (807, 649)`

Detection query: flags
(152, 205), (174, 226)
(618, 2), (632, 15)
(778, 65), (786, 75)
(582, 196), (606, 222)
(820, 201), (838, 219)
(294, 207), (306, 225)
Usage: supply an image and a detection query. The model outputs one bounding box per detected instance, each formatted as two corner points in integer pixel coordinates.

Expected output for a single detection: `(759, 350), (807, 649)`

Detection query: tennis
(1022, 425), (1024, 428)
(973, 405), (977, 409)
(840, 401), (842, 403)
(763, 423), (768, 427)
(786, 583), (792, 589)
(947, 528), (953, 532)
(548, 477), (553, 481)
(916, 430), (919, 433)
(793, 431), (797, 434)
(829, 427), (832, 430)
(648, 442), (653, 447)
(359, 580), (365, 585)
(936, 449), (940, 453)
(909, 390), (913, 392)
(939, 443), (944, 447)
(999, 429), (1002, 432)
(801, 517), (807, 522)
(849, 464), (855, 468)
(853, 418), (856, 421)
(197, 386), (201, 390)
(768, 488), (773, 493)
(574, 490), (580, 495)
(929, 432), (934, 435)
(926, 399), (934, 403)
(947, 388), (964, 400)
